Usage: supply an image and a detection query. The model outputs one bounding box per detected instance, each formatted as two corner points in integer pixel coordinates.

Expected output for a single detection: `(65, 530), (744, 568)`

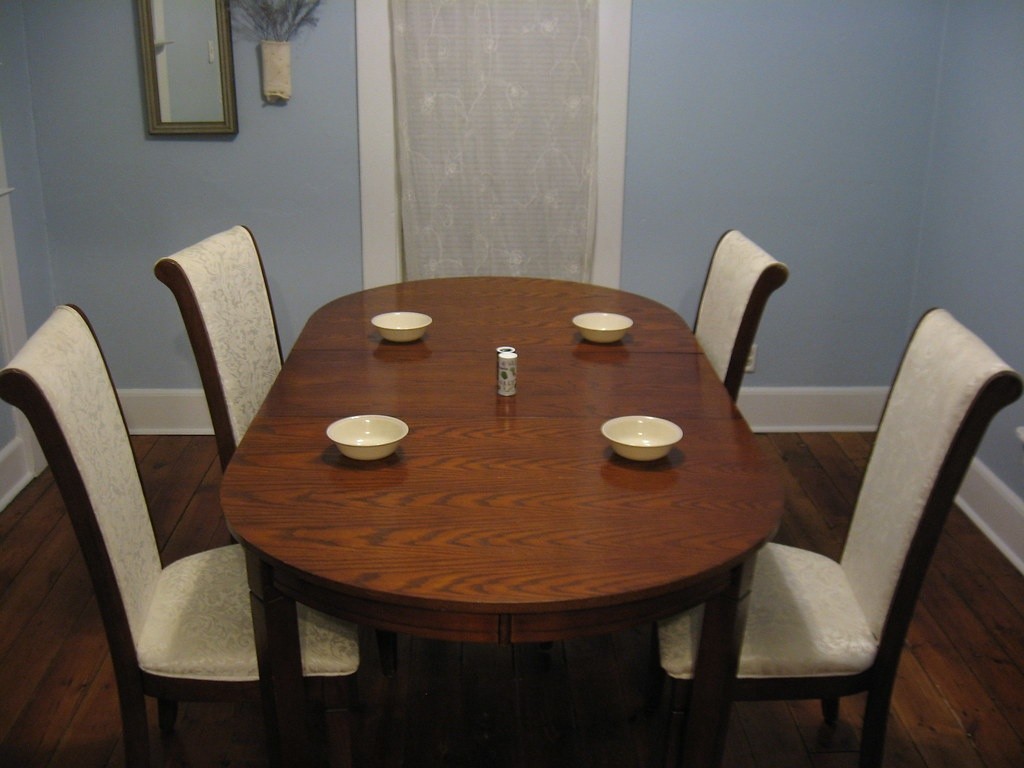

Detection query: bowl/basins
(370, 311), (432, 343)
(571, 312), (634, 343)
(600, 415), (683, 462)
(326, 415), (409, 461)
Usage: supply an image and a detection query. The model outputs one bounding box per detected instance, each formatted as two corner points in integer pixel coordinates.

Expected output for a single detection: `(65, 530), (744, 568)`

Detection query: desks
(215, 275), (785, 767)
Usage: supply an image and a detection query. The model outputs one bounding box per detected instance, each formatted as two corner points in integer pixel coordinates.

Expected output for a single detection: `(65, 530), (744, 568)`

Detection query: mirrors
(136, 0), (239, 135)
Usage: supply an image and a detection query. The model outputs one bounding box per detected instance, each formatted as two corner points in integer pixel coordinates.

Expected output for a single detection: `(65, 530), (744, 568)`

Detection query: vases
(259, 38), (293, 102)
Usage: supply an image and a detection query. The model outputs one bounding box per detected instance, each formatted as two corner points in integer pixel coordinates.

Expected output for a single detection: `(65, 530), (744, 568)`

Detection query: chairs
(152, 225), (396, 680)
(1, 302), (365, 768)
(640, 307), (1023, 768)
(689, 229), (792, 406)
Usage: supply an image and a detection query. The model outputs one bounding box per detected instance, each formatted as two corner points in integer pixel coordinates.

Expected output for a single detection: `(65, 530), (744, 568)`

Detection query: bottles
(496, 346), (518, 396)
